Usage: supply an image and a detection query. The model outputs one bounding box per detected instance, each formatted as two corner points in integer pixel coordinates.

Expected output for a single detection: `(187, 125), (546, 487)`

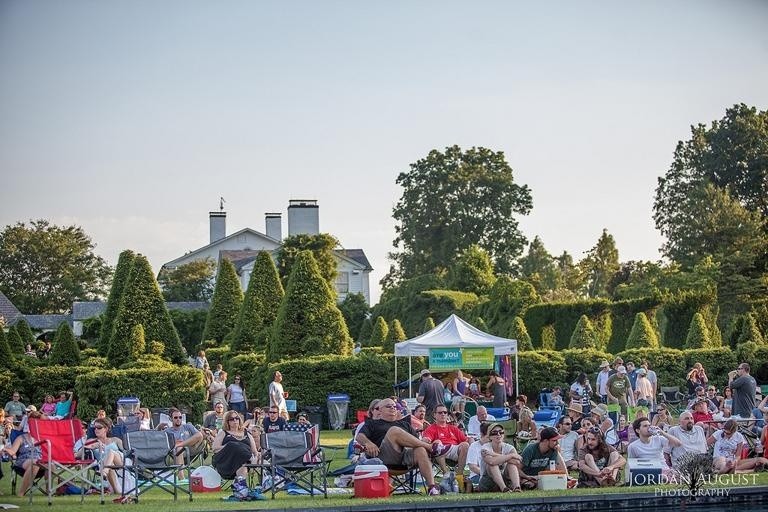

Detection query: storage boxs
(625, 457), (663, 486)
(354, 464), (388, 499)
(538, 474), (567, 491)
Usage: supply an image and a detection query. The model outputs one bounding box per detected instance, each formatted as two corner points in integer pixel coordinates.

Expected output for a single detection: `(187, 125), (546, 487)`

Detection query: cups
(284, 392), (288, 398)
(99, 444), (105, 454)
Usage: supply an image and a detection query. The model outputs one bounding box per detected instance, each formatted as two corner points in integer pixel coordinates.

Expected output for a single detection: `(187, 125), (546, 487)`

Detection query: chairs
(419, 421), (457, 479)
(121, 431), (194, 504)
(260, 431), (327, 500)
(647, 412), (657, 423)
(87, 424), (128, 497)
(541, 394), (553, 410)
(10, 429), (49, 497)
(658, 386), (685, 416)
(531, 406), (561, 428)
(486, 408), (510, 419)
(606, 411), (623, 455)
(363, 415), (429, 497)
(302, 424), (345, 488)
(28, 418), (105, 506)
(169, 439), (208, 466)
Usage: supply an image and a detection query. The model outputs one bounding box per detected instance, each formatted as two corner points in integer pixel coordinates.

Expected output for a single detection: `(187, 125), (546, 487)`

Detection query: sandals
(96, 469), (109, 482)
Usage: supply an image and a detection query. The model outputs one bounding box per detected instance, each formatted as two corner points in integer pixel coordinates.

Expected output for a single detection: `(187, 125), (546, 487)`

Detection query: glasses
(562, 422), (572, 426)
(590, 427), (603, 436)
(434, 411), (448, 414)
(657, 409), (665, 411)
(617, 363), (622, 365)
(95, 425), (106, 430)
(298, 413), (308, 417)
(724, 430), (734, 435)
(173, 416), (183, 419)
(381, 405), (397, 409)
(490, 430), (505, 436)
(698, 394), (705, 397)
(581, 422), (592, 428)
(373, 406), (380, 411)
(737, 367), (746, 370)
(228, 416), (240, 421)
(269, 411), (279, 414)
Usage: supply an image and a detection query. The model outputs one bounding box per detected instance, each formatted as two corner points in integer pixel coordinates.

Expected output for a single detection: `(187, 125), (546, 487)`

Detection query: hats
(565, 404), (586, 415)
(394, 403), (405, 410)
(635, 368), (647, 376)
(708, 385), (716, 390)
(488, 423), (504, 433)
(540, 426), (565, 440)
(25, 405), (37, 412)
(690, 398), (710, 410)
(419, 370), (430, 381)
(598, 361), (611, 368)
(590, 404), (609, 416)
(618, 366), (627, 373)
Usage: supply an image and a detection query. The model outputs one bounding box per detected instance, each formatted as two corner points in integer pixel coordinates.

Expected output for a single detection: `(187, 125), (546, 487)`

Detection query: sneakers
(428, 485), (439, 497)
(432, 442), (452, 458)
(503, 487), (524, 494)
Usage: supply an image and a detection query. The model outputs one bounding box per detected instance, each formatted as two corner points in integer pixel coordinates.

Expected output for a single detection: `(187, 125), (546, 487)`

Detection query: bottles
(553, 395), (556, 401)
(648, 428), (660, 436)
(528, 422), (533, 437)
(576, 387), (579, 394)
(755, 437), (763, 453)
(716, 389), (721, 396)
(453, 479), (458, 493)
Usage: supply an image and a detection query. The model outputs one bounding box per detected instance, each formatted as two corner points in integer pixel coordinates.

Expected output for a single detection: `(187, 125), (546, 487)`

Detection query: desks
(703, 419), (764, 437)
(445, 400), (493, 416)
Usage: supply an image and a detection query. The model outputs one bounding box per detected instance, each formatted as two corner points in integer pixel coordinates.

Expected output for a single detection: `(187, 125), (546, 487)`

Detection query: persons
(421, 404), (474, 477)
(626, 362), (637, 404)
(196, 351), (208, 369)
(577, 427), (626, 487)
(706, 386), (724, 411)
(77, 418), (139, 493)
(413, 405), (431, 436)
(209, 371), (228, 412)
(355, 397), (441, 495)
(203, 402), (224, 439)
(263, 405), (286, 433)
(297, 411), (309, 424)
(269, 370), (289, 421)
(251, 426), (262, 449)
(0, 424), (15, 445)
(706, 420), (756, 474)
(591, 404), (620, 449)
(40, 394), (56, 416)
(3, 412), (54, 498)
(354, 399), (382, 456)
(487, 370), (506, 407)
(566, 404), (584, 434)
(26, 344), (32, 355)
(451, 371), (468, 417)
(686, 368), (701, 401)
(635, 368), (653, 419)
(355, 340), (362, 355)
(520, 427), (577, 488)
(641, 361), (657, 411)
(466, 378), (479, 397)
(45, 391), (73, 420)
(728, 364), (756, 424)
(652, 403), (674, 431)
(577, 419), (598, 456)
(463, 423), (490, 491)
(690, 398), (720, 437)
(556, 416), (580, 471)
(445, 383), (453, 401)
(615, 413), (630, 453)
(44, 341), (52, 354)
(569, 373), (593, 418)
(723, 386), (734, 417)
(516, 395), (535, 434)
(4, 392), (26, 423)
(753, 394), (768, 470)
(694, 362), (708, 392)
(469, 405), (496, 440)
(628, 417), (681, 474)
(595, 361), (613, 404)
(158, 410), (202, 480)
(606, 366), (634, 421)
(479, 423), (523, 493)
(631, 390), (651, 421)
(203, 363), (214, 388)
(227, 375), (249, 421)
(667, 411), (709, 469)
(609, 358), (623, 378)
(214, 364), (225, 379)
(244, 407), (262, 429)
(6, 404), (38, 434)
(548, 386), (566, 411)
(686, 386), (718, 412)
(213, 411), (257, 480)
(417, 369), (445, 421)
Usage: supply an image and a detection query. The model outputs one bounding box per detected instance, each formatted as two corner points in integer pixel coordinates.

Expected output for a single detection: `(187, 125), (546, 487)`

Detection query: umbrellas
(395, 313), (518, 398)
